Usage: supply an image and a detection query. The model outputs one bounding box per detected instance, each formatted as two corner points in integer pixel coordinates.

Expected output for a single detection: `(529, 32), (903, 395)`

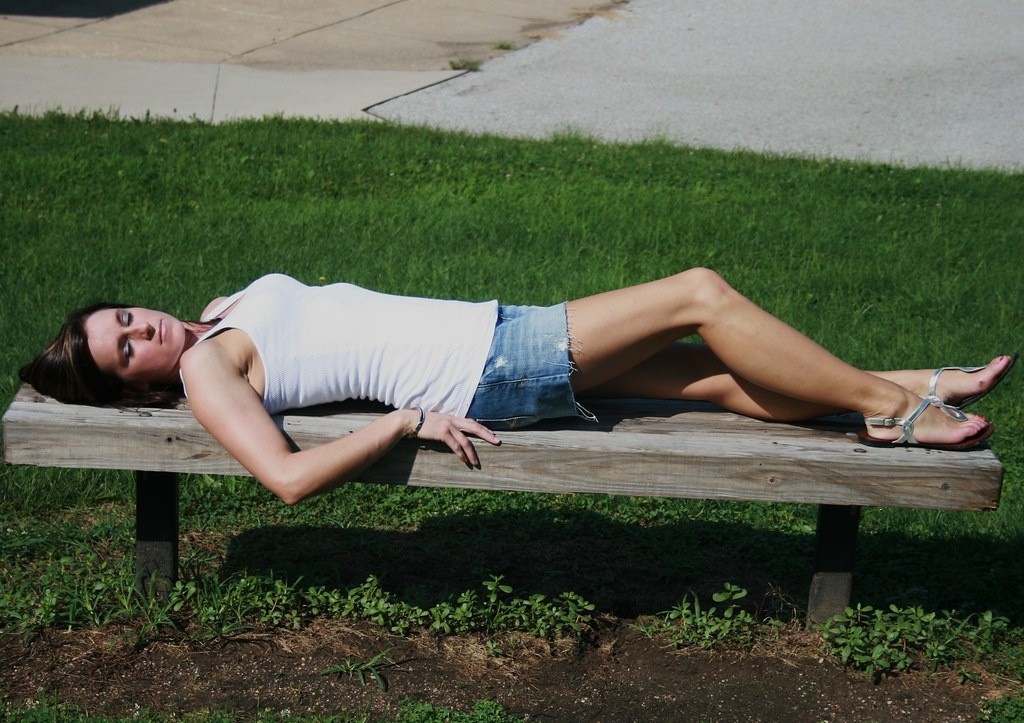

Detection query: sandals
(858, 396), (994, 450)
(925, 355), (1017, 410)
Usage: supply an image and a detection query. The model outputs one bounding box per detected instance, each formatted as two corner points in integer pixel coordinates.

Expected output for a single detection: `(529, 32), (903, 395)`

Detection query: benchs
(2, 376), (1006, 625)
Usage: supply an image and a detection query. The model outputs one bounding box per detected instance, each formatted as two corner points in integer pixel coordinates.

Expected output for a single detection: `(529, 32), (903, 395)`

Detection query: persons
(17, 267), (1019, 506)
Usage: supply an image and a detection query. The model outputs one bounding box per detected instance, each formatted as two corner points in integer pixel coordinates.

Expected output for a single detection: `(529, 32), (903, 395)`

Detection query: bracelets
(409, 404), (426, 437)
